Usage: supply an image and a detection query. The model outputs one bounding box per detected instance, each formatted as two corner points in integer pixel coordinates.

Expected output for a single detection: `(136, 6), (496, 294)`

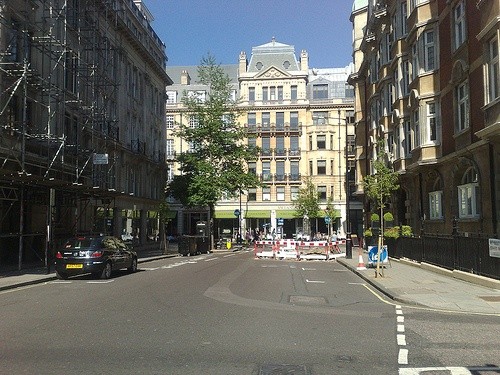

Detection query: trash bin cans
(199, 235), (209, 253)
(188, 236), (200, 255)
(178, 235), (189, 256)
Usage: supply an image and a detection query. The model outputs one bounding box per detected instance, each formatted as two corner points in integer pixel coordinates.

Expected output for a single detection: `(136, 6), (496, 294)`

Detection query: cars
(53, 232), (138, 279)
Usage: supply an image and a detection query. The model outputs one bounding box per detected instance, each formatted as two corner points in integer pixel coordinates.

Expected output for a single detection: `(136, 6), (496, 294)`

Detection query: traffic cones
(356, 250), (368, 271)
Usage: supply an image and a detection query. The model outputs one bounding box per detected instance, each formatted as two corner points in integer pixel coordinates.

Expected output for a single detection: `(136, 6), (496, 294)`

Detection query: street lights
(311, 115), (352, 260)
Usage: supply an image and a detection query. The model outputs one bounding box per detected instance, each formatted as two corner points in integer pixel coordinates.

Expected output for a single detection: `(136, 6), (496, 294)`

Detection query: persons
(330, 231), (337, 242)
